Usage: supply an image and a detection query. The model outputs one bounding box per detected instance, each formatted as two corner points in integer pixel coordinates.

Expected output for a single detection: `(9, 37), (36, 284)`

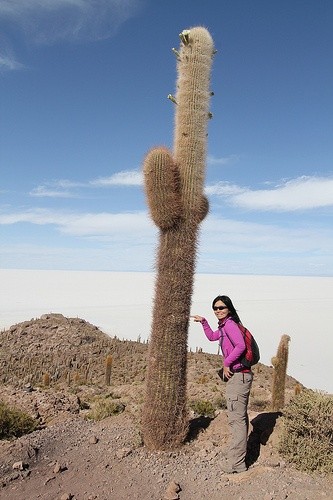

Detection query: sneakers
(221, 461), (248, 473)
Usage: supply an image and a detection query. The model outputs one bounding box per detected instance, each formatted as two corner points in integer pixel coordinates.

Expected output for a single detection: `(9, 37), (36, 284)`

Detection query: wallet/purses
(218, 365), (234, 382)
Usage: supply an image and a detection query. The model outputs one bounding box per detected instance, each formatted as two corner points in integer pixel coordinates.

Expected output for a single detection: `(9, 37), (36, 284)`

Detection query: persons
(188, 294), (255, 474)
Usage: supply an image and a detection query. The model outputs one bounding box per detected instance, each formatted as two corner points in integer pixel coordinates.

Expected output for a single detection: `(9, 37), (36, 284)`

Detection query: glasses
(213, 306), (229, 312)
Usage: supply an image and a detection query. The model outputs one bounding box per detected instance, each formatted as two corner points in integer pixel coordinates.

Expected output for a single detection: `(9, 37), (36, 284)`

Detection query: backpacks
(223, 319), (260, 370)
